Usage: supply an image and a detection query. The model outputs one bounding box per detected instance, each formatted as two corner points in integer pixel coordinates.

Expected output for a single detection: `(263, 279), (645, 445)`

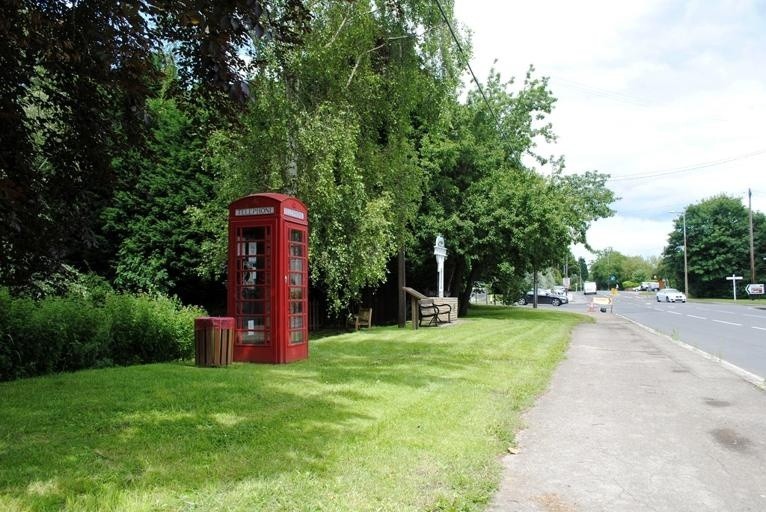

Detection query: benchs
(418, 298), (451, 327)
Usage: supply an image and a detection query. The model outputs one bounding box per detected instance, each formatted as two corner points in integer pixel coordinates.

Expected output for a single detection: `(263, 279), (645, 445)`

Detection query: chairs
(346, 306), (373, 330)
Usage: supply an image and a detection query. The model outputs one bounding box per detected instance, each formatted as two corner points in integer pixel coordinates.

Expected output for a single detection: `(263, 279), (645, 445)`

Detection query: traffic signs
(745, 283), (765, 295)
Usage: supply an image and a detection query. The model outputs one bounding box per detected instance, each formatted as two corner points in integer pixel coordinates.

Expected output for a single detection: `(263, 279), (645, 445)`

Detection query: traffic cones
(586, 300), (596, 312)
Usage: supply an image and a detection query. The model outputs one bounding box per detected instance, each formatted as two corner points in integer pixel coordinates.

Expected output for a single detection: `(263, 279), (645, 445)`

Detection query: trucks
(641, 281), (660, 292)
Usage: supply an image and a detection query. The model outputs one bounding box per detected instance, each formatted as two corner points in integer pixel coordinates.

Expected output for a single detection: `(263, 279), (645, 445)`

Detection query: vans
(582, 281), (597, 295)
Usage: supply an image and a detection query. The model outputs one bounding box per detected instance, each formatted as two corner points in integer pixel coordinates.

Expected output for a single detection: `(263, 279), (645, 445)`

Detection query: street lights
(668, 208), (688, 299)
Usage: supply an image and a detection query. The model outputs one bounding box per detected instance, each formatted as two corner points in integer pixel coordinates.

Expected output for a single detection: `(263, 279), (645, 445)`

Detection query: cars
(656, 288), (687, 303)
(632, 285), (642, 292)
(518, 287), (568, 306)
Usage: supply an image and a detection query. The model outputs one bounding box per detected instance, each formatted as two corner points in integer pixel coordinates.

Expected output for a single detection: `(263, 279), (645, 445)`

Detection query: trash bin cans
(194, 317), (236, 368)
